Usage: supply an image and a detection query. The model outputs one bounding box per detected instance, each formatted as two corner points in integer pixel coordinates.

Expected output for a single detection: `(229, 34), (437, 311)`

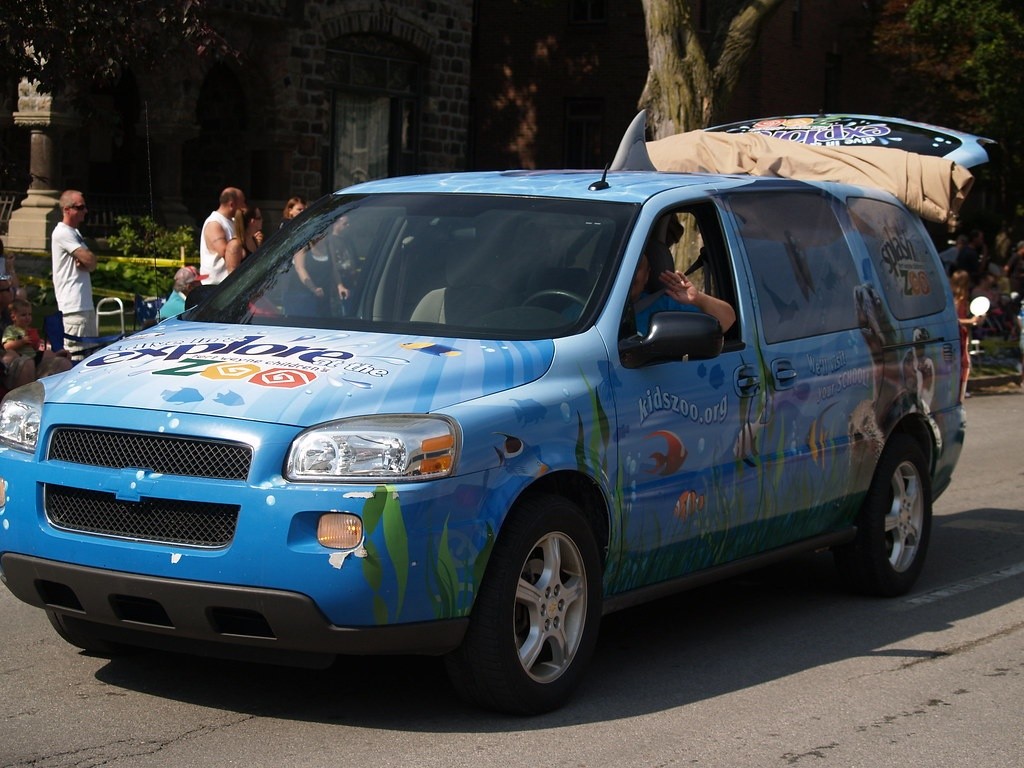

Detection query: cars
(1, 112), (997, 725)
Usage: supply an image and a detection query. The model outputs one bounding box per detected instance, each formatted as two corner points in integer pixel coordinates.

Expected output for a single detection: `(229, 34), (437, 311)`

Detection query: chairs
(127, 295), (166, 334)
(644, 244), (676, 294)
(42, 311), (125, 363)
(411, 243), (507, 323)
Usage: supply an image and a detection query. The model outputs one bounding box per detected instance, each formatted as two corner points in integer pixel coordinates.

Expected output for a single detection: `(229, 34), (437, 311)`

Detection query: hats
(174, 266), (209, 284)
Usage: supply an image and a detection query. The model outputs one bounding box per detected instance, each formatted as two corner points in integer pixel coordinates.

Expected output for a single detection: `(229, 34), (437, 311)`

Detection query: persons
(0, 276), (72, 395)
(51, 191), (97, 360)
(200, 188), (278, 318)
(155, 267), (209, 323)
(279, 197), (362, 318)
(561, 251), (736, 331)
(0, 298), (40, 373)
(953, 271), (987, 398)
(940, 231), (1024, 387)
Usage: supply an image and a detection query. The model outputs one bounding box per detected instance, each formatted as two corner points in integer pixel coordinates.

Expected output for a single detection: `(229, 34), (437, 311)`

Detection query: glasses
(69, 204), (88, 211)
(0, 285), (13, 293)
(255, 216), (262, 220)
